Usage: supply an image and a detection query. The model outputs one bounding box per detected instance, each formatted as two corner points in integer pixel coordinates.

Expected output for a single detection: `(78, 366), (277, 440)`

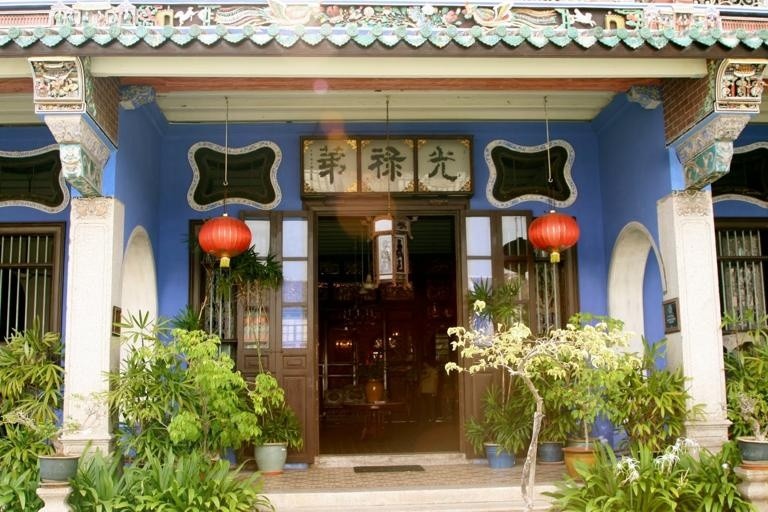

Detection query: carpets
(354, 465), (426, 474)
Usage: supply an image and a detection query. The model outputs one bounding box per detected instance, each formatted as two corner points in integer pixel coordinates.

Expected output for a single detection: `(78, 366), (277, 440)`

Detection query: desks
(346, 401), (405, 444)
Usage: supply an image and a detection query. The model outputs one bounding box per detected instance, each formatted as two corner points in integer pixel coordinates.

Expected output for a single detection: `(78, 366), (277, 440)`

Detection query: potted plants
(462, 371), (604, 485)
(250, 402), (305, 475)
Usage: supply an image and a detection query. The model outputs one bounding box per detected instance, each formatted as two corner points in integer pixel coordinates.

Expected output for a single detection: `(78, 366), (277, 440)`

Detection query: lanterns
(528, 210), (579, 265)
(358, 214), (419, 296)
(198, 212), (251, 268)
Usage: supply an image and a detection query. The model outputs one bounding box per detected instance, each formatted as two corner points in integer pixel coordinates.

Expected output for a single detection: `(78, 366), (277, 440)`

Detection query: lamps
(360, 93), (419, 293)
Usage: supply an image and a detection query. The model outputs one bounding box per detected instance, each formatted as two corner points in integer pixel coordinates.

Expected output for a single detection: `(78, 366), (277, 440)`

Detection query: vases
(38, 455), (80, 484)
(366, 378), (382, 403)
(737, 436), (768, 471)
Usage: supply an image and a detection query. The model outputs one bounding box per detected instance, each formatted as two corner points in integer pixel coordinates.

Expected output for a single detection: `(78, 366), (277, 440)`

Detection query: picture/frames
(112, 305), (121, 338)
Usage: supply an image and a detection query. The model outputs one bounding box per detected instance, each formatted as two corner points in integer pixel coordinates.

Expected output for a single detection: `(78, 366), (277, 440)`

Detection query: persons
(413, 359), (438, 424)
